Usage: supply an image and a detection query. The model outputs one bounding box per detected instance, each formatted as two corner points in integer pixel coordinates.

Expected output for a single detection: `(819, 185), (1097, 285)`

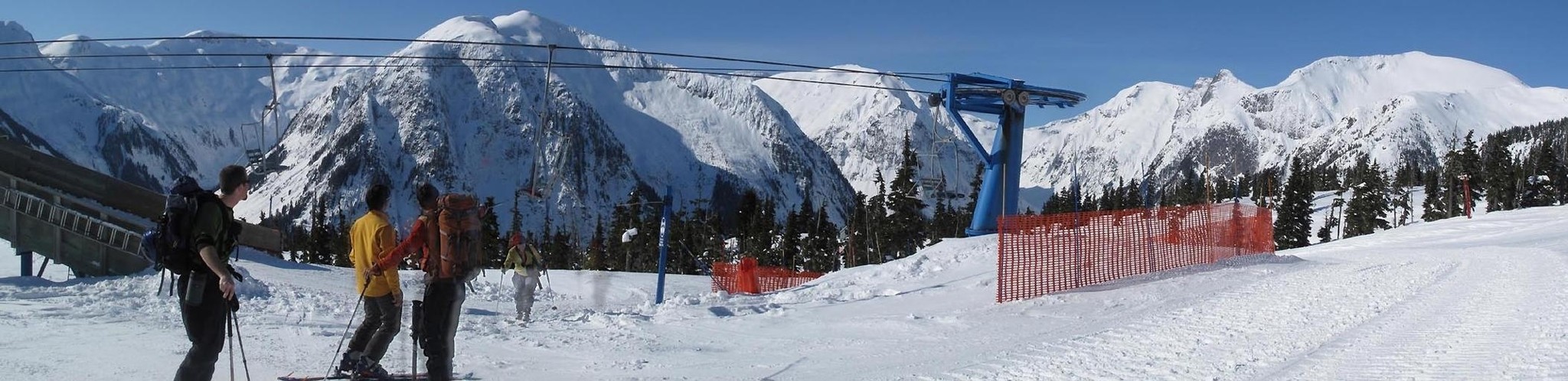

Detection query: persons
(339, 184), (466, 381)
(501, 233), (544, 321)
(174, 165), (249, 381)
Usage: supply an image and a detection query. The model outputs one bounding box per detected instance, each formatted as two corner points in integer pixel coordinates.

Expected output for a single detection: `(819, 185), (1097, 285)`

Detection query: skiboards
(504, 318), (536, 331)
(277, 371), (478, 381)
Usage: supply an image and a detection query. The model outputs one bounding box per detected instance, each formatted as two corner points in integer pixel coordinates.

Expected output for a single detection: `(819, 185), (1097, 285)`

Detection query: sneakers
(356, 354), (388, 377)
(340, 352), (361, 371)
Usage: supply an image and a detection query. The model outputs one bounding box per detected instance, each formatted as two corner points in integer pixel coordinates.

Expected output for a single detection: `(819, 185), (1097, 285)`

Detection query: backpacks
(140, 176), (230, 273)
(424, 191), (486, 285)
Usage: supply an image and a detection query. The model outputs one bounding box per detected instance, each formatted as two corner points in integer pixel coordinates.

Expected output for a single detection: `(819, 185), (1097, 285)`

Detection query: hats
(512, 234), (527, 244)
